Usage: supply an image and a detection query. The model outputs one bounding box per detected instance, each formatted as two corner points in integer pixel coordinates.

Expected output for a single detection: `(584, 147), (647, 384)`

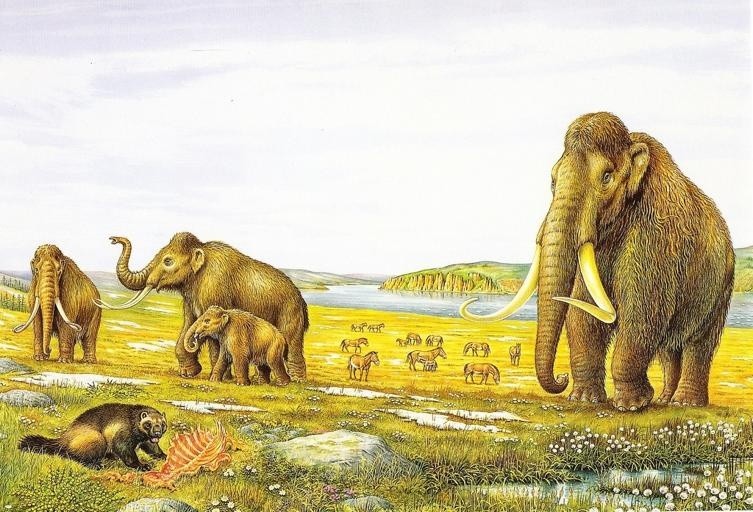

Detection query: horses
(463, 362), (501, 385)
(463, 341), (491, 358)
(339, 337), (369, 354)
(367, 323), (385, 333)
(346, 350), (381, 382)
(509, 342), (521, 367)
(395, 330), (447, 372)
(350, 322), (368, 332)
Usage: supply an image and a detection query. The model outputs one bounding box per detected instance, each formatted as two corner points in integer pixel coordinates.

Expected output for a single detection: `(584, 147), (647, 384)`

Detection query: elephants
(458, 110), (736, 412)
(11, 244), (102, 365)
(89, 231), (310, 387)
